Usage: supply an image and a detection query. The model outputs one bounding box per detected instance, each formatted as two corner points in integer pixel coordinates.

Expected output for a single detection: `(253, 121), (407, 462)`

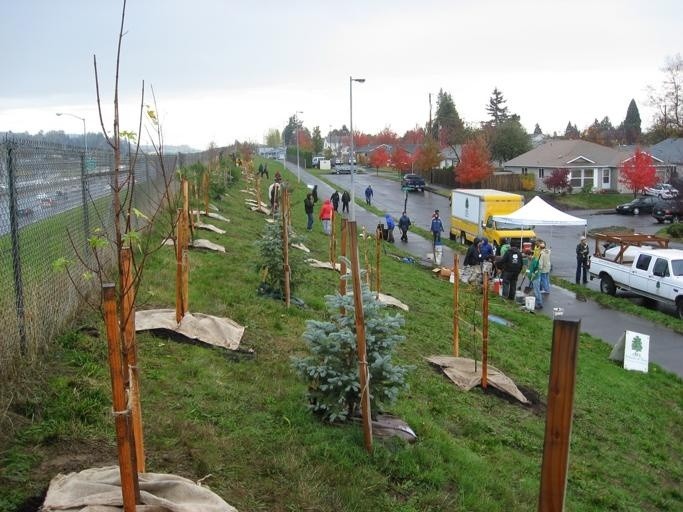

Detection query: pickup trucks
(641, 183), (678, 200)
(589, 249), (683, 321)
(400, 174), (425, 192)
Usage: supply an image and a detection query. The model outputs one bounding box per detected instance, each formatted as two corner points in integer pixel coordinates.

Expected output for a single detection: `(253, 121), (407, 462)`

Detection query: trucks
(448, 189), (536, 256)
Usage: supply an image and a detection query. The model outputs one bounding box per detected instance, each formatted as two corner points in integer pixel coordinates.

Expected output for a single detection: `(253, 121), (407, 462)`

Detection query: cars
(86, 165), (128, 177)
(616, 196), (663, 215)
(332, 168), (355, 176)
(334, 158), (343, 165)
(348, 157), (356, 165)
(16, 184), (79, 220)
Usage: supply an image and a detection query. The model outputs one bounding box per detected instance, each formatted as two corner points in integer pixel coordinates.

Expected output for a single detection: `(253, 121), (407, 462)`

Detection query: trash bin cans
(378, 224), (389, 240)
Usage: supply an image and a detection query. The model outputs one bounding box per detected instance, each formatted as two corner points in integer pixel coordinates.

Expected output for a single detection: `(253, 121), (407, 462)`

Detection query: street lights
(348, 77), (366, 222)
(282, 119), (289, 170)
(55, 113), (87, 151)
(295, 110), (305, 183)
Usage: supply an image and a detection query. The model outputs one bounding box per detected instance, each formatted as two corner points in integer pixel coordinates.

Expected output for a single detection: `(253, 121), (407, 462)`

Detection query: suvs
(652, 201), (683, 223)
(311, 157), (326, 169)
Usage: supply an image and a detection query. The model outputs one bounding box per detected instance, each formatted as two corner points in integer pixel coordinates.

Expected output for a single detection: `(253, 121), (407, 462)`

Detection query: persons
(574, 234), (591, 285)
(255, 162), (263, 177)
(341, 190), (350, 214)
(383, 208), (551, 309)
(268, 171), (294, 209)
(260, 163), (269, 180)
(329, 190), (339, 212)
(303, 193), (314, 232)
(236, 158), (243, 175)
(364, 185), (373, 206)
(311, 185), (317, 205)
(318, 199), (334, 235)
